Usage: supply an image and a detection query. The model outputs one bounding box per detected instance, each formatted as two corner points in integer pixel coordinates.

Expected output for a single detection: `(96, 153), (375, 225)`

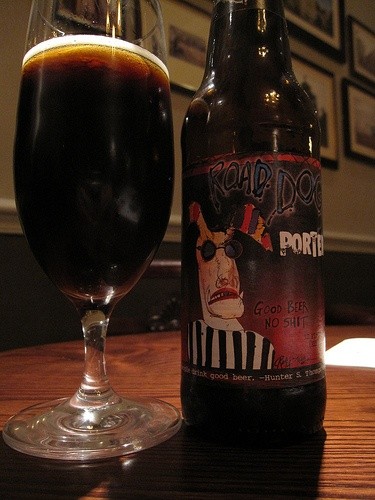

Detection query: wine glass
(2, 0), (182, 461)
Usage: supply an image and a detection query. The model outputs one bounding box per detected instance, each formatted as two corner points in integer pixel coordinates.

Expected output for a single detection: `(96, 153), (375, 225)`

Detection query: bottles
(181, 0), (329, 442)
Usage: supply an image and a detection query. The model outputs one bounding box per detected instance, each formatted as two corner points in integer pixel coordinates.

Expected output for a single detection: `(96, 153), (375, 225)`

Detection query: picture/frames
(134, 1), (213, 97)
(349, 15), (375, 83)
(53, 1), (126, 39)
(342, 77), (375, 164)
(289, 50), (340, 170)
(284, 0), (346, 65)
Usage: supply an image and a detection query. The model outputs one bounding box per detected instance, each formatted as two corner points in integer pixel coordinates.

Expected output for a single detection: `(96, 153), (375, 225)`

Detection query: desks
(1, 325), (375, 500)
